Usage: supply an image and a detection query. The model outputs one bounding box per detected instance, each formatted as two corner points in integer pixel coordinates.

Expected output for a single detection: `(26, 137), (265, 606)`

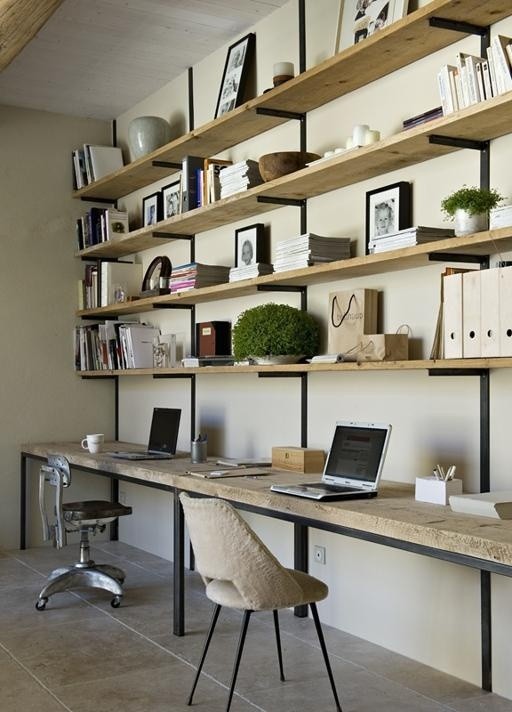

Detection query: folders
(443, 266), (512, 360)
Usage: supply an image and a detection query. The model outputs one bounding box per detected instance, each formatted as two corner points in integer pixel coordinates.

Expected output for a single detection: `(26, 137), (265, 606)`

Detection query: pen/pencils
(192, 433), (208, 442)
(185, 471), (207, 478)
(433, 464), (456, 481)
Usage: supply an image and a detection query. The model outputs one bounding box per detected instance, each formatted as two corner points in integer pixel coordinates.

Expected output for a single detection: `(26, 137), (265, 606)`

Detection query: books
(168, 260), (272, 296)
(400, 32), (512, 136)
(74, 259), (146, 311)
(75, 204), (131, 252)
(272, 223), (455, 275)
(74, 317), (234, 372)
(69, 140), (125, 193)
(179, 154), (265, 215)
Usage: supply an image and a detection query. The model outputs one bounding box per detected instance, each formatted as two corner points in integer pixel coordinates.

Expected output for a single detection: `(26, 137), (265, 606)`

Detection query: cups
(273, 62), (295, 88)
(323, 125), (381, 158)
(191, 440), (208, 464)
(81, 434), (105, 454)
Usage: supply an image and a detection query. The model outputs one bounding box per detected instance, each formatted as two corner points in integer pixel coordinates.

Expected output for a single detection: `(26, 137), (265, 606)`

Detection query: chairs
(27, 447), (140, 610)
(178, 484), (344, 712)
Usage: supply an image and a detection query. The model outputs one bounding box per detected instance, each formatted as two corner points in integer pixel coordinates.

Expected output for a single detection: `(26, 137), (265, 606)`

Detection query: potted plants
(438, 186), (501, 235)
(228, 301), (325, 370)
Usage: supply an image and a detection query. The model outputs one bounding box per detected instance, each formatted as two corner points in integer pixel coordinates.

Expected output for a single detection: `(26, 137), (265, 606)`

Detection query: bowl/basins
(258, 152), (321, 182)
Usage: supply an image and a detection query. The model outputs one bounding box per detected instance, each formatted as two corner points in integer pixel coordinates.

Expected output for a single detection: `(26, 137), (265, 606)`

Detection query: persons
(147, 204), (156, 226)
(218, 46), (246, 117)
(241, 238), (253, 266)
(167, 190), (179, 220)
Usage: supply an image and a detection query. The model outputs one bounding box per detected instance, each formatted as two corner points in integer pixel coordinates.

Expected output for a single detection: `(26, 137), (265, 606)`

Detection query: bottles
(126, 114), (172, 161)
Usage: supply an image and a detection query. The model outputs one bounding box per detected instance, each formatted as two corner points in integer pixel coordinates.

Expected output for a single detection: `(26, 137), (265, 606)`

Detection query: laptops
(106, 407), (182, 460)
(270, 421), (392, 501)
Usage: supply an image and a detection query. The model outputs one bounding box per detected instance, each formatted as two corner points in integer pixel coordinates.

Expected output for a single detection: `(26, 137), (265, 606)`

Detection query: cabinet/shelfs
(68, 0), (510, 385)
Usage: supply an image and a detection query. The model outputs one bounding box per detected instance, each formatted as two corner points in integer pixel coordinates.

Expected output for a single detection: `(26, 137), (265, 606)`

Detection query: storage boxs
(271, 442), (327, 475)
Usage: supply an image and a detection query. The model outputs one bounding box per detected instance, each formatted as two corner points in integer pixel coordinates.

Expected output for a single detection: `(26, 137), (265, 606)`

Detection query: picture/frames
(361, 178), (414, 255)
(138, 188), (162, 224)
(230, 222), (264, 275)
(213, 31), (255, 118)
(159, 177), (185, 218)
(333, 1), (410, 56)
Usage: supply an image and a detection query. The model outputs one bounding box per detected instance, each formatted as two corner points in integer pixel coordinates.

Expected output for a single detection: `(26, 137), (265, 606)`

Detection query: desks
(173, 469), (509, 697)
(20, 431), (218, 577)
